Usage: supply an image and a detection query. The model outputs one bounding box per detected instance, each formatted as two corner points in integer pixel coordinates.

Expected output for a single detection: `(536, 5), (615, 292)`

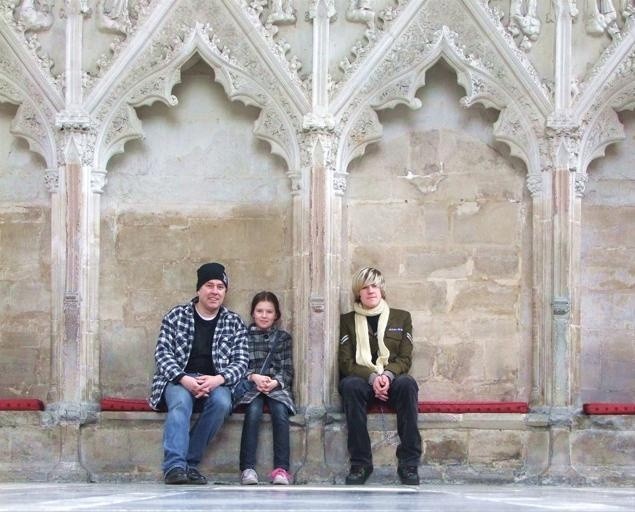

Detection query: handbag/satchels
(231, 377), (256, 403)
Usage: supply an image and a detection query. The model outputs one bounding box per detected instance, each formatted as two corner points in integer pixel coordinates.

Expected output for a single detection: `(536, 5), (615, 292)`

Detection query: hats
(196, 263), (228, 292)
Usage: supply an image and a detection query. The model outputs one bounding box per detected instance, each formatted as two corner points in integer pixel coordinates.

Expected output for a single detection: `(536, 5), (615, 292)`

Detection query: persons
(230, 290), (296, 485)
(147, 262), (250, 484)
(338, 266), (423, 486)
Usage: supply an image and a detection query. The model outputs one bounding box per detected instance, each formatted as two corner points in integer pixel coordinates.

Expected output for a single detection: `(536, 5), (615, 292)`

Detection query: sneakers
(165, 467), (187, 484)
(188, 468), (207, 484)
(346, 464), (373, 484)
(271, 468), (292, 484)
(397, 464), (419, 484)
(241, 469), (258, 484)
